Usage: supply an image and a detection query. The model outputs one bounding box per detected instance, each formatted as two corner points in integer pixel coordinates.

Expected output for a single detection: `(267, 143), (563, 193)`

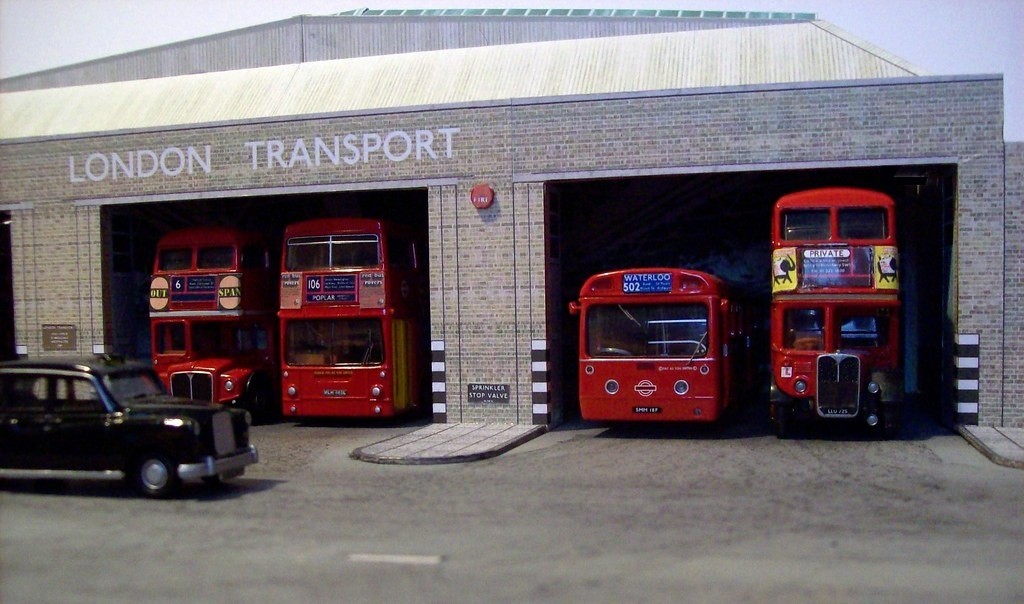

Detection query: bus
(567, 266), (765, 423)
(278, 218), (429, 417)
(148, 227), (279, 414)
(768, 186), (902, 429)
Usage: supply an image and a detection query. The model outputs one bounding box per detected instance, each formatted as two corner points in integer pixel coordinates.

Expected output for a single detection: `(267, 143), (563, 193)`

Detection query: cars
(0, 357), (258, 500)
